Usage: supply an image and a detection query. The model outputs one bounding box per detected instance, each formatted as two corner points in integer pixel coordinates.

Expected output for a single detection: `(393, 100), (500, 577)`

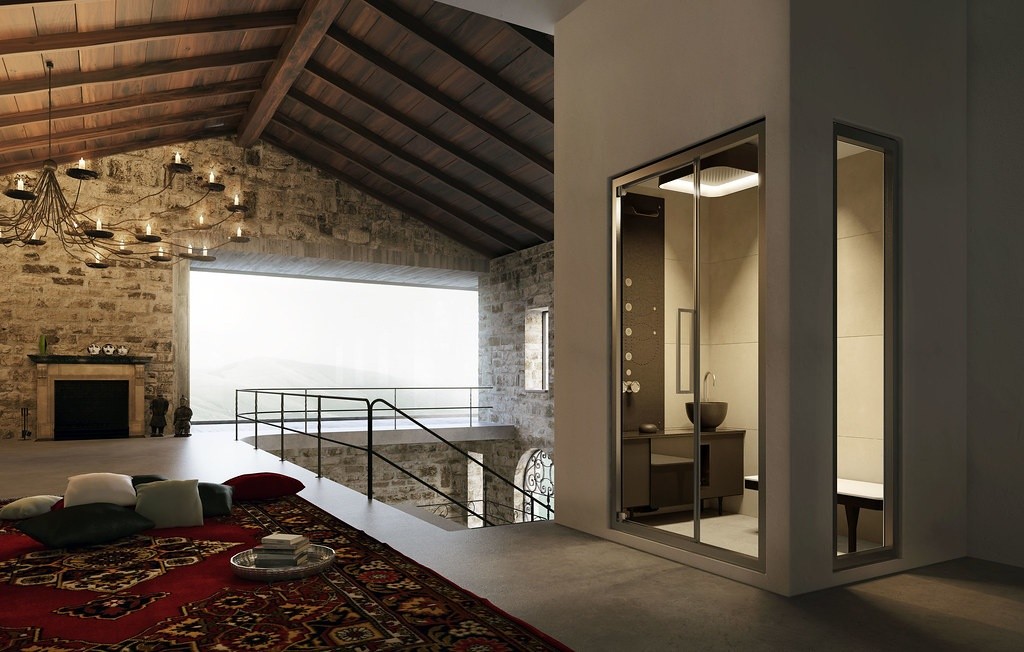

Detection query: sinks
(685, 401), (728, 432)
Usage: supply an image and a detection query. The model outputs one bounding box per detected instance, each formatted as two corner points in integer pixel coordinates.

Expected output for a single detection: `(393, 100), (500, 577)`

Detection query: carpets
(0, 497), (578, 652)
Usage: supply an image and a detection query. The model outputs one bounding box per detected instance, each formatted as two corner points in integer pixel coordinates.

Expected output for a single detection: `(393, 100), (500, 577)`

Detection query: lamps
(658, 143), (759, 199)
(0, 61), (251, 268)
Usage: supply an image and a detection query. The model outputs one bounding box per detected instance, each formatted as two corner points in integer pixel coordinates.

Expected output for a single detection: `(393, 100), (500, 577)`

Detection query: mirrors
(677, 308), (694, 393)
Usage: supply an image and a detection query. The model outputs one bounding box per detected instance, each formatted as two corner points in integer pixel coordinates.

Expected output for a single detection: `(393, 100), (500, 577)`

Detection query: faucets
(702, 371), (716, 402)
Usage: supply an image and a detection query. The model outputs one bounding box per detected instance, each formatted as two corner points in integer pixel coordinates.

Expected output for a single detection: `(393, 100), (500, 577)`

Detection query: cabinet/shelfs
(621, 428), (746, 520)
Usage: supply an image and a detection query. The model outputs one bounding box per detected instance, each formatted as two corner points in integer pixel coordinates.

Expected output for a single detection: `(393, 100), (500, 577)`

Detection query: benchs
(745, 475), (885, 555)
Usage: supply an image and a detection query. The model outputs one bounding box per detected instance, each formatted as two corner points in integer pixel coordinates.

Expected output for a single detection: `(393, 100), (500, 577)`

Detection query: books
(253, 533), (311, 569)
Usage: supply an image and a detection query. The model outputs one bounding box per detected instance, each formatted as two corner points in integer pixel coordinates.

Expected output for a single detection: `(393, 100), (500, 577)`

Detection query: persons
(172, 393), (195, 438)
(148, 392), (171, 438)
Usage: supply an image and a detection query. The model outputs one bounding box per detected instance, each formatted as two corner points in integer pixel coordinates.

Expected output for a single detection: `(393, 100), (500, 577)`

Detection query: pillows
(0, 472), (305, 551)
